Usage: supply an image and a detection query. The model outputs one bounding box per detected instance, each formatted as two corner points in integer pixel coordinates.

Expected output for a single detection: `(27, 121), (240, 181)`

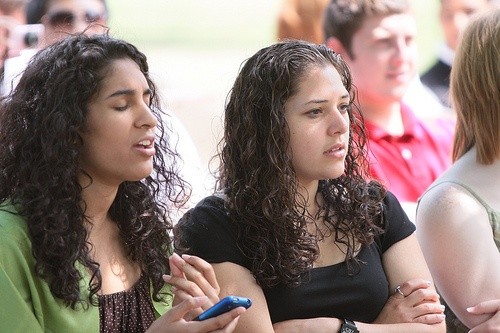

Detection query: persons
(0, 0), (500, 228)
(173, 40), (448, 333)
(415, 8), (500, 333)
(0, 35), (247, 333)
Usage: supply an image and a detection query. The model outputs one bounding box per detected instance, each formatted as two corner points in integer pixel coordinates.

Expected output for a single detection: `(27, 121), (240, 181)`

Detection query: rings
(395, 285), (406, 298)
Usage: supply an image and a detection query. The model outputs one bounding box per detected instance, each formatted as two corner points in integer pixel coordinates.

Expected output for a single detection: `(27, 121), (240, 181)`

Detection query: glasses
(39, 11), (106, 29)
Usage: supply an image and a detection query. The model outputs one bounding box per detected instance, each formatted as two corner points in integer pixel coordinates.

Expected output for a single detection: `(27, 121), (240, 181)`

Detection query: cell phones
(192, 295), (252, 321)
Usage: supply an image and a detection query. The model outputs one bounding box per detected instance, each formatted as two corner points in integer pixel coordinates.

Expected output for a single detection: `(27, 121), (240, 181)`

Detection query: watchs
(340, 317), (360, 333)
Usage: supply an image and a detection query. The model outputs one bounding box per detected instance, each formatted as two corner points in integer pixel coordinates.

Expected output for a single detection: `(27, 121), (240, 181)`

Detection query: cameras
(5, 23), (46, 55)
